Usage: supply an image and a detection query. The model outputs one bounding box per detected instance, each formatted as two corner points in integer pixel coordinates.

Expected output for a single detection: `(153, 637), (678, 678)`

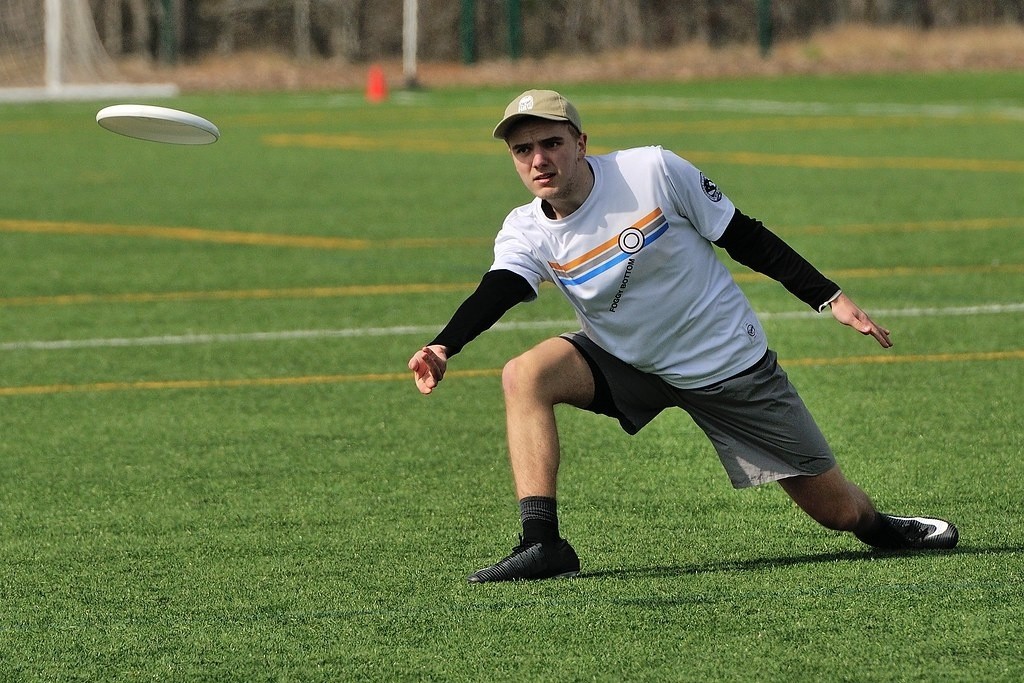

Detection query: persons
(408, 88), (959, 584)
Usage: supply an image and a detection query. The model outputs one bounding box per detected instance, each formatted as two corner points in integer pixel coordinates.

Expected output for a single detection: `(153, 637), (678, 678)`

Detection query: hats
(494, 89), (581, 145)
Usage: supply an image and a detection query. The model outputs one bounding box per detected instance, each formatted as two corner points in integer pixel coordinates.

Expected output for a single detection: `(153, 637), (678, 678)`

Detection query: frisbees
(96, 104), (221, 146)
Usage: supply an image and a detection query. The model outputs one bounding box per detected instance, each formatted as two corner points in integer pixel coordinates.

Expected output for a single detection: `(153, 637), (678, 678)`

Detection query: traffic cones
(365, 66), (390, 104)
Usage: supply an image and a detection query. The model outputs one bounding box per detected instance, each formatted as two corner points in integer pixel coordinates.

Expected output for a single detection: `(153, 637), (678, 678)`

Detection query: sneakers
(861, 512), (959, 550)
(467, 533), (580, 584)
